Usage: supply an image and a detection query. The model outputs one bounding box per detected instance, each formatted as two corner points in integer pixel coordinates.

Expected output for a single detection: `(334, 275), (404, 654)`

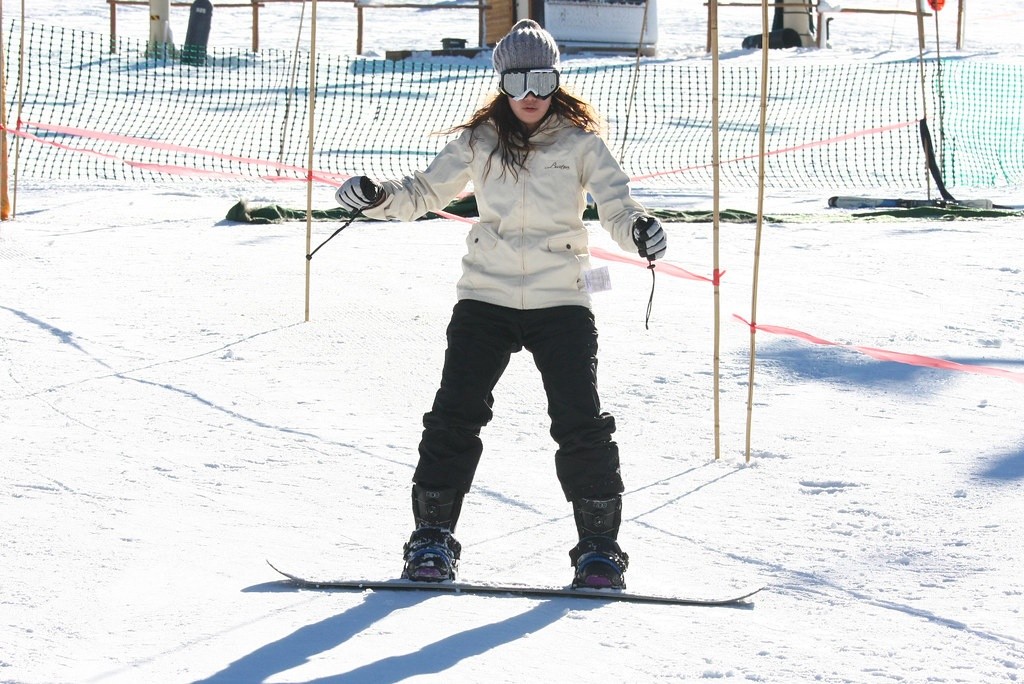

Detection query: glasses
(496, 68), (560, 100)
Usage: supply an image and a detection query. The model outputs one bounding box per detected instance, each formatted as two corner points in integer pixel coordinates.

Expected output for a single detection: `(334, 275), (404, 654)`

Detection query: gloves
(335, 176), (383, 212)
(633, 215), (667, 261)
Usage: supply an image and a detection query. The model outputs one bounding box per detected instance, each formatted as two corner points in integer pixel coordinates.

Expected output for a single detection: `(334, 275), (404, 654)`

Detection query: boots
(572, 496), (617, 586)
(410, 485), (464, 579)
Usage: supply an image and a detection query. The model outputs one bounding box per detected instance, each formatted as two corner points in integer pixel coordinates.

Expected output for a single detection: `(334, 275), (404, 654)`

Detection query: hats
(492, 19), (560, 73)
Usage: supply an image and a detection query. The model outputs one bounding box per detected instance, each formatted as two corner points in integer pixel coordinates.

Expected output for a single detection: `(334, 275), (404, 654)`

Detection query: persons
(335, 18), (670, 594)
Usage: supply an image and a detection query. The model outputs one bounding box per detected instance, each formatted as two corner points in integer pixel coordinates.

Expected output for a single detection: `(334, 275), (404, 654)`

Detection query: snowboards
(266, 557), (765, 607)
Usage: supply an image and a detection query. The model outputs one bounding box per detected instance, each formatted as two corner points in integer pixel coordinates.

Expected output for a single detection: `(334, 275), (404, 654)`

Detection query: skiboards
(828, 196), (994, 211)
